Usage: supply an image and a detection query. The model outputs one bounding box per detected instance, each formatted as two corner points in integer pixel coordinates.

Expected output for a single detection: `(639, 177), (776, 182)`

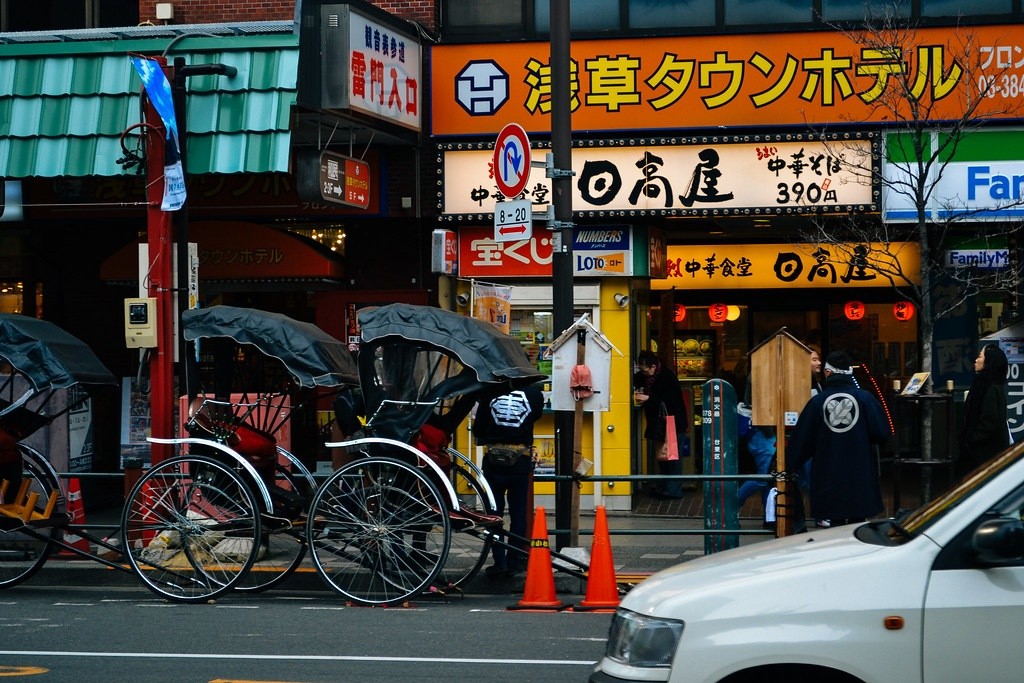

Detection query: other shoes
(658, 494), (682, 500)
(816, 519), (830, 528)
(485, 560), (524, 576)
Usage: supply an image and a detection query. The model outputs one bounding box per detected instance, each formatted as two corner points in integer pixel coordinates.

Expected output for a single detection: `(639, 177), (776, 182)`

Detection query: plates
(673, 339), (684, 353)
(684, 339), (700, 355)
(699, 340), (713, 355)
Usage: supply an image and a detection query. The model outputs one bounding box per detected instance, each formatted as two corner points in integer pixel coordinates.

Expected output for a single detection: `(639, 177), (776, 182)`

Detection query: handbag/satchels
(487, 446), (519, 468)
(644, 402), (679, 460)
(736, 402), (752, 436)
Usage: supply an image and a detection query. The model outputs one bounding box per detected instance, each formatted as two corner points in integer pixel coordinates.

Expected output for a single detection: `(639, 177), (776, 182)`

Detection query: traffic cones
(138, 473), (158, 549)
(581, 505), (622, 614)
(56, 478), (95, 556)
(509, 506), (576, 612)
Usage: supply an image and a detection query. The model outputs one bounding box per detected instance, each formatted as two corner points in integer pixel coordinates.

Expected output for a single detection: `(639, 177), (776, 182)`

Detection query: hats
(826, 351), (852, 370)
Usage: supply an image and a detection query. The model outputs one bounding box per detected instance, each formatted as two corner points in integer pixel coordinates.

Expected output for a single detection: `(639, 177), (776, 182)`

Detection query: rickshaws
(0, 311), (211, 597)
(305, 304), (635, 607)
(120, 304), (464, 606)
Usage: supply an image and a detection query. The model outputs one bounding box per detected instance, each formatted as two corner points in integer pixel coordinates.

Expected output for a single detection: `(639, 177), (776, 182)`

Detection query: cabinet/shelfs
(674, 356), (708, 406)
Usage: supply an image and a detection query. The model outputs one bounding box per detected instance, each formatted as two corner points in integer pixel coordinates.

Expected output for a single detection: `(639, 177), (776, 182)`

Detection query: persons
(385, 382), (439, 569)
(956, 343), (1010, 487)
(473, 373), (543, 580)
(736, 371), (776, 513)
(777, 345), (889, 529)
(635, 349), (689, 499)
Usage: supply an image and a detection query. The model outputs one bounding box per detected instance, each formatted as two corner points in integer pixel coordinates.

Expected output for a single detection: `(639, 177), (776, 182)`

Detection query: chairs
(196, 403), (277, 456)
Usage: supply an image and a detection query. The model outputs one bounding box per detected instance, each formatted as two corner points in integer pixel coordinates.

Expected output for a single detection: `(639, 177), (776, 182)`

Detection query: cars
(586, 436), (1024, 683)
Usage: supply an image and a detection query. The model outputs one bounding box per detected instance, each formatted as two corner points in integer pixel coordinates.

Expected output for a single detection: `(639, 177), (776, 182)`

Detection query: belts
(483, 444), (531, 456)
